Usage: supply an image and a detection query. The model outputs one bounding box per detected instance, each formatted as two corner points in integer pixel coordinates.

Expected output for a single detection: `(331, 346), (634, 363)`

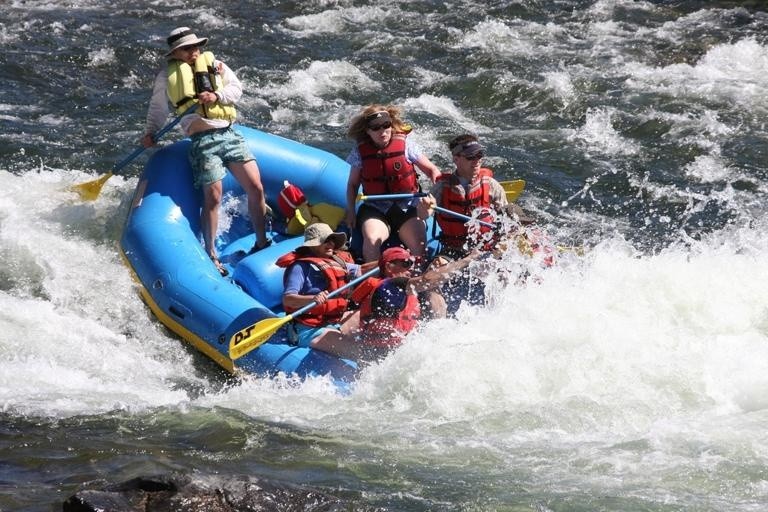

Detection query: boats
(117, 124), (592, 403)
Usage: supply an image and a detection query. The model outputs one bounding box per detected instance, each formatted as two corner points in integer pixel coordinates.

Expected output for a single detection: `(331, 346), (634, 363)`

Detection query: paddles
(66, 103), (201, 202)
(356, 179), (526, 204)
(228, 266), (381, 361)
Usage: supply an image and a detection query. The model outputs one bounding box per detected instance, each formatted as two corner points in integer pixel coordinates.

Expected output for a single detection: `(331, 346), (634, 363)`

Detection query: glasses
(371, 121), (391, 131)
(463, 152), (483, 160)
(401, 260), (413, 268)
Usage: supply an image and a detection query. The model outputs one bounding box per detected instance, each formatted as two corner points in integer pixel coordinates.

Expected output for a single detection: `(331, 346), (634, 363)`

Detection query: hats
(163, 25), (209, 57)
(378, 247), (415, 269)
(364, 110), (393, 129)
(295, 222), (347, 254)
(451, 141), (487, 156)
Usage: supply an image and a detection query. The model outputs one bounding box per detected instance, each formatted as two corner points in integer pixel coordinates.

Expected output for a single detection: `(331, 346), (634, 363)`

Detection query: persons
(347, 105), (442, 265)
(418, 135), (509, 272)
(351, 247), (484, 357)
(143, 25), (272, 277)
(276, 223), (379, 360)
